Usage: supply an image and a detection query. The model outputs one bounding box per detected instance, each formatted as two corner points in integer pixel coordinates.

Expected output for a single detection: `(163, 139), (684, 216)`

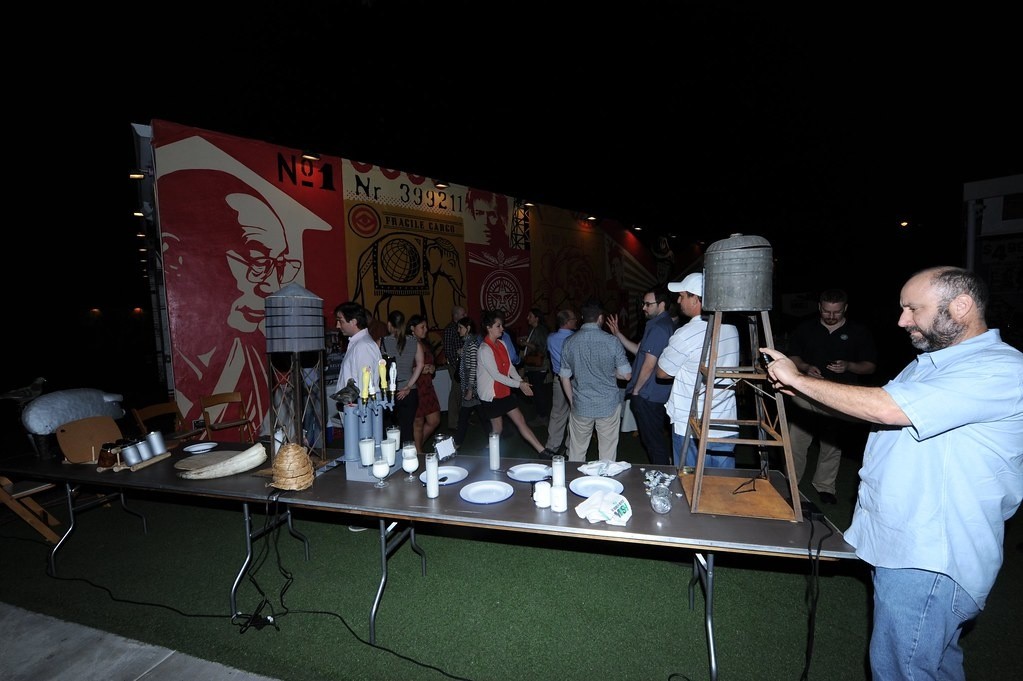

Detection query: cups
(651, 483), (672, 514)
(121, 429), (167, 467)
(533, 481), (550, 509)
(550, 487), (567, 512)
(359, 437), (375, 467)
(380, 425), (401, 467)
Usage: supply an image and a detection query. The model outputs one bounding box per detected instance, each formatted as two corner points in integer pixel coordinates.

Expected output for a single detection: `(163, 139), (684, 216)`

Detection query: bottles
(426, 452), (440, 498)
(489, 431), (499, 469)
(552, 455), (565, 487)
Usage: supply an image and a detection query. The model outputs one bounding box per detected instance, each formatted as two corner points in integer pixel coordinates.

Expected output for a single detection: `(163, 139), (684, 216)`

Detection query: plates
(508, 463), (552, 482)
(419, 466), (468, 485)
(460, 480), (514, 504)
(183, 442), (218, 454)
(570, 475), (624, 498)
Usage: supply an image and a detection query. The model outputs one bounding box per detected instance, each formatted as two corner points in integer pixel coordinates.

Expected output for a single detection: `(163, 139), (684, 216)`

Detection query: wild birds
(329, 378), (360, 406)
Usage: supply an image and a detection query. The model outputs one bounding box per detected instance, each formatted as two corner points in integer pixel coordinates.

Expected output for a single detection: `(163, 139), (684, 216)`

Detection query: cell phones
(757, 353), (778, 386)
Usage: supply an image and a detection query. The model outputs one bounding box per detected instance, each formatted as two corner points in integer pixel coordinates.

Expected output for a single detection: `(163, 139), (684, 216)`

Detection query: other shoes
(537, 448), (569, 462)
(817, 491), (837, 505)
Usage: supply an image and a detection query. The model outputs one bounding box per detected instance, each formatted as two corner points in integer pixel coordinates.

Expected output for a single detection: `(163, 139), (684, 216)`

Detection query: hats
(667, 273), (703, 297)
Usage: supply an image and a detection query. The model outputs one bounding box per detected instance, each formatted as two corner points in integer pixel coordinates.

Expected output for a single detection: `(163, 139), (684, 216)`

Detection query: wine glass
(402, 440), (419, 482)
(373, 456), (390, 488)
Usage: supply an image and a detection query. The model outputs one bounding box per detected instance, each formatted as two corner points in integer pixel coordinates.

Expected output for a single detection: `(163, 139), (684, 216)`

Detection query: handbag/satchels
(379, 337), (397, 381)
(523, 354), (543, 368)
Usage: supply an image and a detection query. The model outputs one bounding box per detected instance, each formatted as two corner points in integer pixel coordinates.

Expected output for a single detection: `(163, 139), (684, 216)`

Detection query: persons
(477, 309), (551, 426)
(475, 311), (558, 460)
(334, 301), (382, 427)
(785, 288), (877, 504)
(657, 272), (739, 468)
(546, 310), (577, 460)
(558, 306), (632, 462)
(605, 288), (676, 464)
(759, 266), (1023, 681)
(371, 305), (492, 453)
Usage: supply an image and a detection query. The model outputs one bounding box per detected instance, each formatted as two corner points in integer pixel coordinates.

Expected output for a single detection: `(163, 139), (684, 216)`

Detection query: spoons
(423, 476), (448, 486)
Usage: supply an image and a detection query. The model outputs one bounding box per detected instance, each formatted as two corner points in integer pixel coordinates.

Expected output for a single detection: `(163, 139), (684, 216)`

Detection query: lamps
(523, 200), (535, 207)
(435, 180), (451, 189)
(130, 167), (154, 179)
(301, 149), (320, 161)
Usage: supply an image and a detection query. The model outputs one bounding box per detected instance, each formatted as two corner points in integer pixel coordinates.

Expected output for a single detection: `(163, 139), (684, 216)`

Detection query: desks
(277, 454), (862, 680)
(0, 438), (343, 624)
(325, 367), (453, 444)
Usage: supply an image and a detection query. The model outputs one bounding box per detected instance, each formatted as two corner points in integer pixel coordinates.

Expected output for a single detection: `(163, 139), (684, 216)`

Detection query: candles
(533, 480), (568, 512)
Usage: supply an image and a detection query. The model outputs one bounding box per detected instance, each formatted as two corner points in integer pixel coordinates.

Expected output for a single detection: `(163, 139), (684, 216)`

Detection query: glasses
(641, 301), (659, 308)
(819, 307), (844, 316)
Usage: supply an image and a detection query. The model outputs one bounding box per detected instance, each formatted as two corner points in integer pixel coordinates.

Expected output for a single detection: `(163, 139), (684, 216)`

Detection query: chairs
(0, 410), (125, 542)
(199, 390), (255, 445)
(131, 398), (203, 443)
